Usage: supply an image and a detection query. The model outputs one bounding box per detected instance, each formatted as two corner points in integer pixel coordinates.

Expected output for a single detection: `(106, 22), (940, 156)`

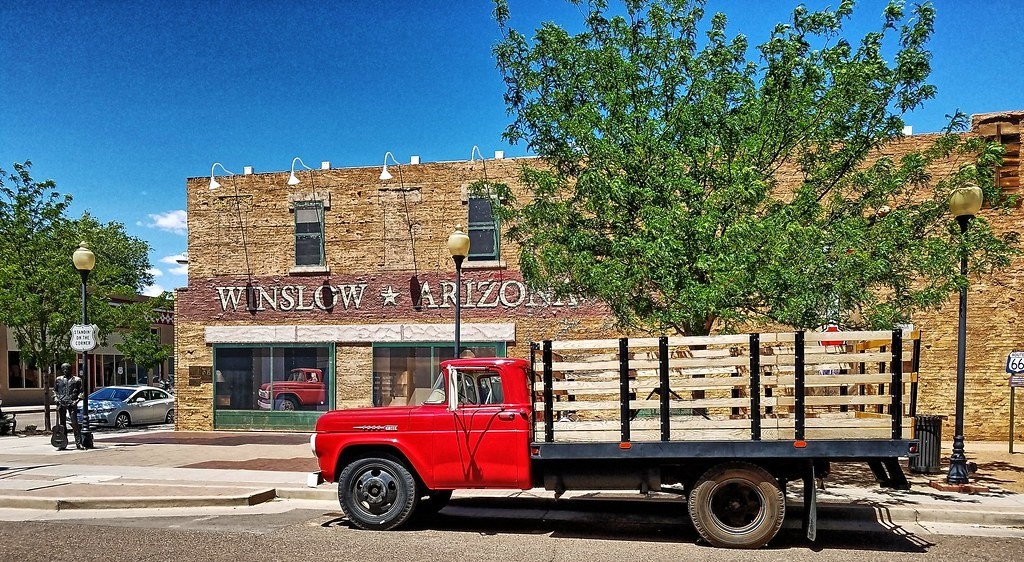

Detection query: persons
(51, 363), (87, 451)
(306, 372), (318, 383)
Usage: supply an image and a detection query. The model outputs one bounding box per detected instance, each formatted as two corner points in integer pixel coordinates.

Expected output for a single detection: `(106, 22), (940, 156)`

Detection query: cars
(67, 384), (176, 430)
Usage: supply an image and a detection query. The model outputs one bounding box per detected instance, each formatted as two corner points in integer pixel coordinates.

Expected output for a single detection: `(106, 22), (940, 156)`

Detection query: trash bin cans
(906, 415), (948, 473)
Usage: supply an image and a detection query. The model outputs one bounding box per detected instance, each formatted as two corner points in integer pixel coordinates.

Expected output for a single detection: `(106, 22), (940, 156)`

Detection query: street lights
(948, 180), (985, 485)
(70, 239), (95, 450)
(446, 222), (471, 359)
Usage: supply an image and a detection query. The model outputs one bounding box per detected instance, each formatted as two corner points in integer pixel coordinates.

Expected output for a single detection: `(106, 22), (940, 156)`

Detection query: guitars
(50, 405), (68, 448)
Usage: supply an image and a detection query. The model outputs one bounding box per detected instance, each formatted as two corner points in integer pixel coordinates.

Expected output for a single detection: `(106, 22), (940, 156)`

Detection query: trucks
(254, 368), (396, 411)
(309, 322), (924, 552)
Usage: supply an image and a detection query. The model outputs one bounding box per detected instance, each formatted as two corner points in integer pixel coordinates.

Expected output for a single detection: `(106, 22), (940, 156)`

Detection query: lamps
(287, 157), (310, 185)
(379, 151), (400, 179)
(209, 162), (234, 190)
(468, 146), (485, 172)
